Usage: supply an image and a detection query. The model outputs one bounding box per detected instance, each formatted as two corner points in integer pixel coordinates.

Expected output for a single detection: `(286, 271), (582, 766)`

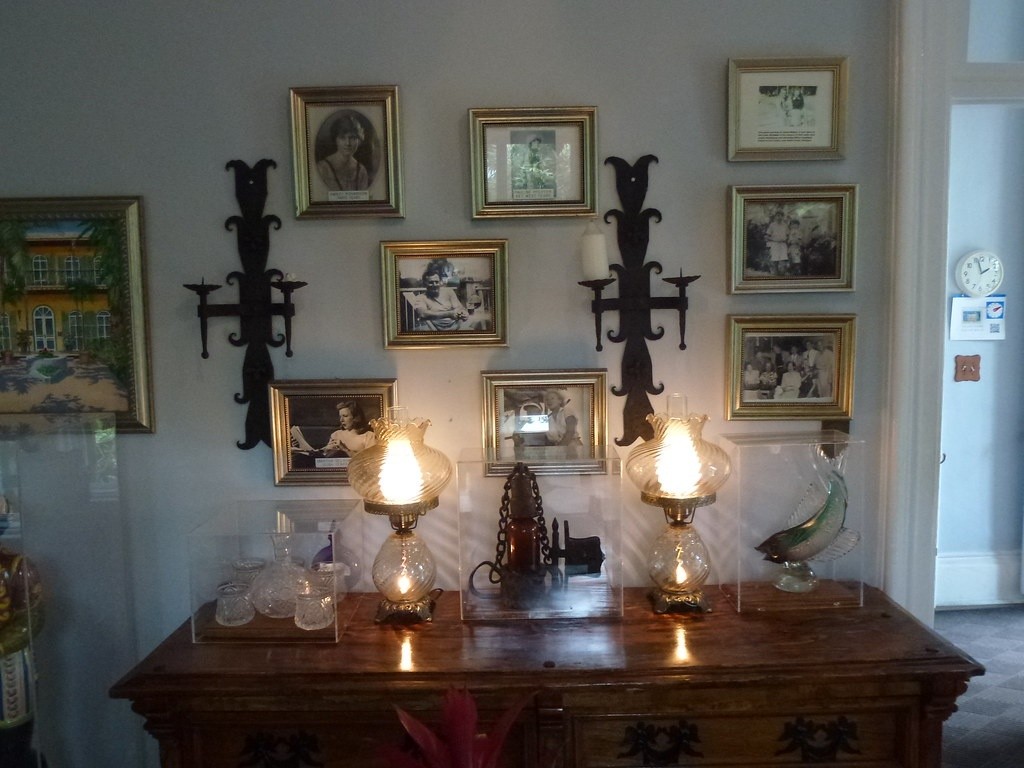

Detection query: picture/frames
(0, 196), (153, 437)
(467, 106), (599, 217)
(376, 238), (508, 347)
(725, 185), (856, 291)
(725, 312), (856, 421)
(478, 370), (612, 475)
(267, 379), (397, 484)
(288, 85), (404, 224)
(726, 57), (848, 163)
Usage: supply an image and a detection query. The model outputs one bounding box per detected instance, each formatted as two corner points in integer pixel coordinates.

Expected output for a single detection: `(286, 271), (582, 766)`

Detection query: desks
(109, 581), (984, 768)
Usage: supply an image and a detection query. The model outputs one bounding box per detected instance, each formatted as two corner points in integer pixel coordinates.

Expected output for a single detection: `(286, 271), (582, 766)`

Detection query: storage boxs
(716, 428), (867, 610)
(456, 446), (627, 622)
(186, 498), (368, 646)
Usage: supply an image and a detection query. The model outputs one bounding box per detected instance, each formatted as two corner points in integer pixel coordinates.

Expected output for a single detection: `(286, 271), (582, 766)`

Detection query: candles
(346, 415), (450, 506)
(582, 231), (611, 279)
(625, 416), (731, 498)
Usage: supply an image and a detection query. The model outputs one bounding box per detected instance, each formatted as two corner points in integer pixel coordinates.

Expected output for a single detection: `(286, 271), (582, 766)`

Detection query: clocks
(955, 251), (1005, 296)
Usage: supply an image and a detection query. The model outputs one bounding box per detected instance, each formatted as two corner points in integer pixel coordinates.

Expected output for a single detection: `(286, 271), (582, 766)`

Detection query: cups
(295, 587), (334, 630)
(216, 580), (256, 627)
(231, 557), (266, 586)
(310, 561), (347, 604)
(271, 558), (305, 578)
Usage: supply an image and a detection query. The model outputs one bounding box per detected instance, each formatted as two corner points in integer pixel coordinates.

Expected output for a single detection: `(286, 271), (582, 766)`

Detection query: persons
(513, 390), (585, 455)
(744, 339), (834, 401)
(290, 402), (377, 458)
(315, 115), (368, 191)
(761, 210), (810, 275)
(414, 270), (471, 330)
